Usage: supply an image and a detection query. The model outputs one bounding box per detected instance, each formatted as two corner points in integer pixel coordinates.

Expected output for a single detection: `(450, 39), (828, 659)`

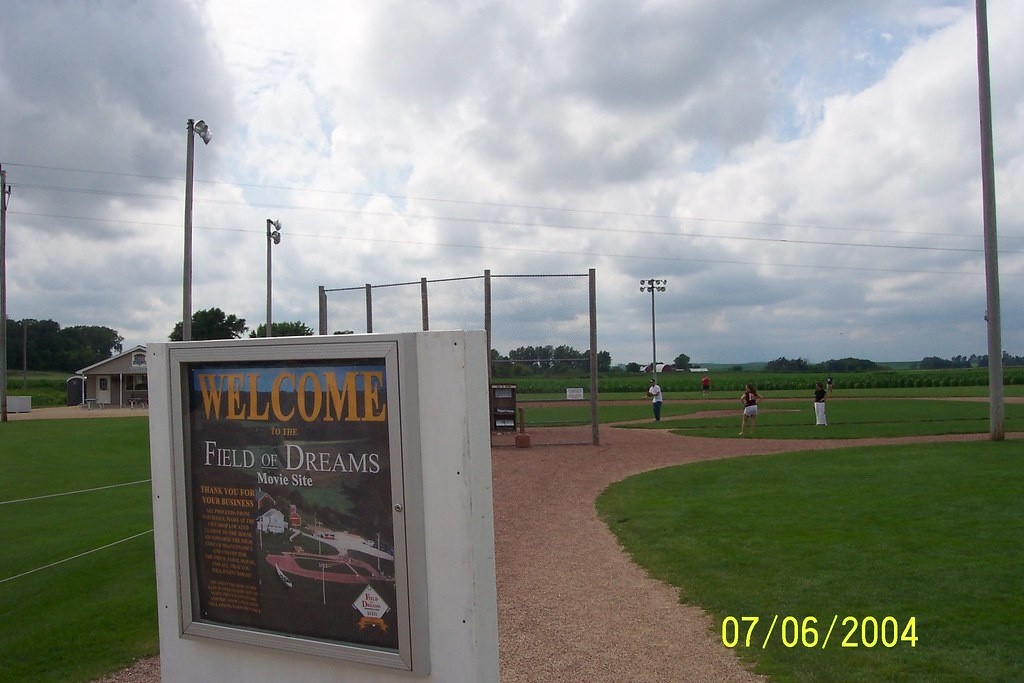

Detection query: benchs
(99, 402), (109, 409)
(79, 403), (87, 409)
(141, 401), (148, 408)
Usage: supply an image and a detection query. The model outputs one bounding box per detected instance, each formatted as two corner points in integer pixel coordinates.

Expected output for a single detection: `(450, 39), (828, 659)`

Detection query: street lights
(264, 217), (284, 340)
(181, 114), (214, 344)
(639, 276), (668, 385)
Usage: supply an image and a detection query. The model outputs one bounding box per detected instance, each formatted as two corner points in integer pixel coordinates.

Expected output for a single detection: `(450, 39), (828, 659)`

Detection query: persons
(814, 373), (833, 427)
(738, 384), (763, 436)
(702, 374), (710, 397)
(642, 379), (663, 423)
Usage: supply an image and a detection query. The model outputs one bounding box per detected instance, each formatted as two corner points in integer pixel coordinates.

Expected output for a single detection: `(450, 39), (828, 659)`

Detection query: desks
(127, 397), (143, 410)
(85, 398), (97, 410)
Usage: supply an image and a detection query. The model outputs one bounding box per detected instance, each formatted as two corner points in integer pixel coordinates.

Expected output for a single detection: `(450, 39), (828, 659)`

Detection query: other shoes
(739, 432), (743, 435)
